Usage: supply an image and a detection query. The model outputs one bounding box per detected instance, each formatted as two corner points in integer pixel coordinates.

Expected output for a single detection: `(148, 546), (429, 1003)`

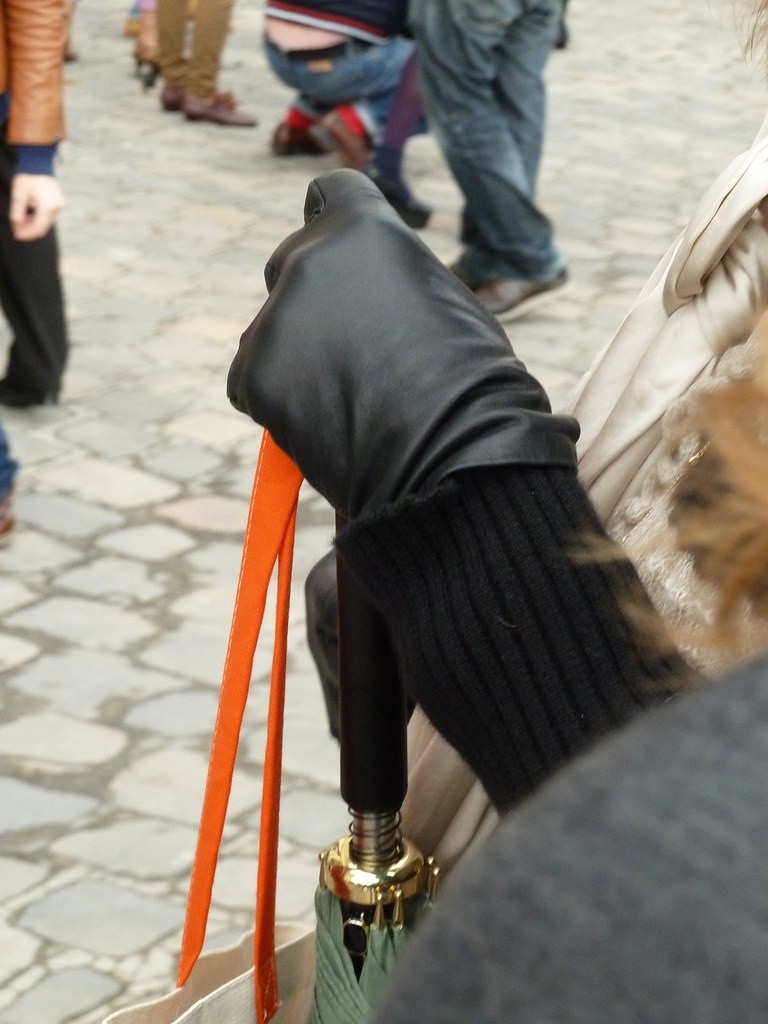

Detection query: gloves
(226, 167), (581, 521)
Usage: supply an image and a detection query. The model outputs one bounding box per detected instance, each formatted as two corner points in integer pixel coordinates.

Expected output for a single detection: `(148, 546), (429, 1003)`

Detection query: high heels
(0, 375), (61, 407)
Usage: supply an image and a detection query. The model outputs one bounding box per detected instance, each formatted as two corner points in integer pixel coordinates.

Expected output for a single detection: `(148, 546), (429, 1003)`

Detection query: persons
(403, 4), (768, 894)
(226, 167), (768, 1023)
(1, 0), (77, 536)
(125, 0), (570, 317)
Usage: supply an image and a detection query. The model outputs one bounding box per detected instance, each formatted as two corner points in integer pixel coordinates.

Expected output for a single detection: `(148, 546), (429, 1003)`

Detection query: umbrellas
(301, 544), (433, 1022)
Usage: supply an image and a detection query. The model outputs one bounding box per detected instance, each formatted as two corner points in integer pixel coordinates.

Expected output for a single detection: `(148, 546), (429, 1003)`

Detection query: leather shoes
(273, 124), (324, 155)
(309, 111), (376, 175)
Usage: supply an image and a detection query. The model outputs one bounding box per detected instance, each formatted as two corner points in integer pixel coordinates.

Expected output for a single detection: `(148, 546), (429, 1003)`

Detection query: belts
(266, 38), (373, 61)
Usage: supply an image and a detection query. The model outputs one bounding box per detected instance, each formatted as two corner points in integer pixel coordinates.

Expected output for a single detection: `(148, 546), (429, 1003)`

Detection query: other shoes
(162, 86), (256, 127)
(124, 19), (140, 37)
(363, 162), (430, 228)
(136, 60), (160, 87)
(0, 483), (16, 547)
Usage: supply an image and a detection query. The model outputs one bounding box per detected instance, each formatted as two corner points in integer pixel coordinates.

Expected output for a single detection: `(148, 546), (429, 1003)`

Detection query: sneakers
(449, 264), (573, 323)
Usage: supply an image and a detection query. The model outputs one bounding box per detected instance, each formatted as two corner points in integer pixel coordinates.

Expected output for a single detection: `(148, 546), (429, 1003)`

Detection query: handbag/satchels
(102, 921), (317, 1024)
